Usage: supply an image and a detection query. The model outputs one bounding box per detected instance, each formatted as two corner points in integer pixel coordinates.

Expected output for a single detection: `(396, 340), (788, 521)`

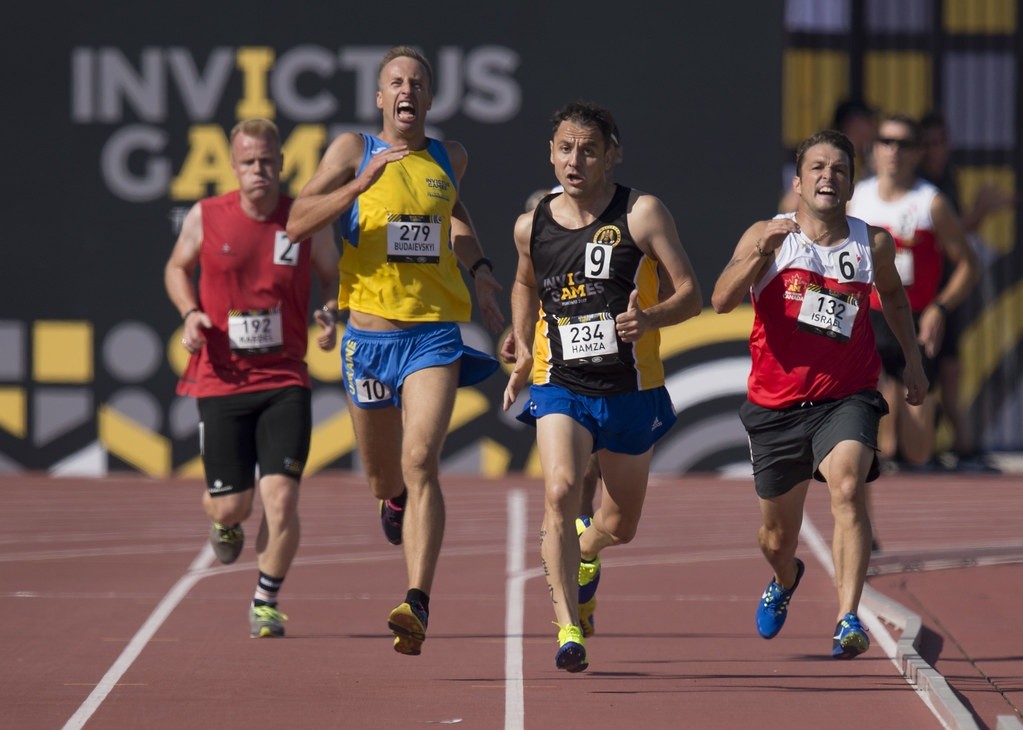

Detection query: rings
(622, 330), (625, 337)
(181, 339), (187, 343)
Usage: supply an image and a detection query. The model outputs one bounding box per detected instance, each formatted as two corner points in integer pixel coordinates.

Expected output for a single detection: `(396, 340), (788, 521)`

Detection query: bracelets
(319, 306), (339, 323)
(757, 237), (774, 256)
(185, 306), (200, 319)
(468, 258), (492, 278)
(935, 300), (952, 317)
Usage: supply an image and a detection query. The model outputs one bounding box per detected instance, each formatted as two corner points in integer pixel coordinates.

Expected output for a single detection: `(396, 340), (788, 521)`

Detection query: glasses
(876, 136), (916, 148)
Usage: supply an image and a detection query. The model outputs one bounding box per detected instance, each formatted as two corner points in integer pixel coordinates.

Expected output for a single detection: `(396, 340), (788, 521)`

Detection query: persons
(163, 119), (339, 637)
(499, 99), (703, 672)
(848, 115), (975, 477)
(711, 126), (929, 659)
(286, 45), (506, 656)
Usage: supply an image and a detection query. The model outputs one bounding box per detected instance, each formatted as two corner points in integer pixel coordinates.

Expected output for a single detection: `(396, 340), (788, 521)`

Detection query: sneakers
(832, 611), (869, 657)
(380, 498), (405, 545)
(574, 516), (601, 604)
(551, 621), (588, 672)
(211, 523), (245, 565)
(249, 601), (288, 637)
(755, 557), (805, 640)
(579, 597), (596, 637)
(387, 600), (428, 655)
